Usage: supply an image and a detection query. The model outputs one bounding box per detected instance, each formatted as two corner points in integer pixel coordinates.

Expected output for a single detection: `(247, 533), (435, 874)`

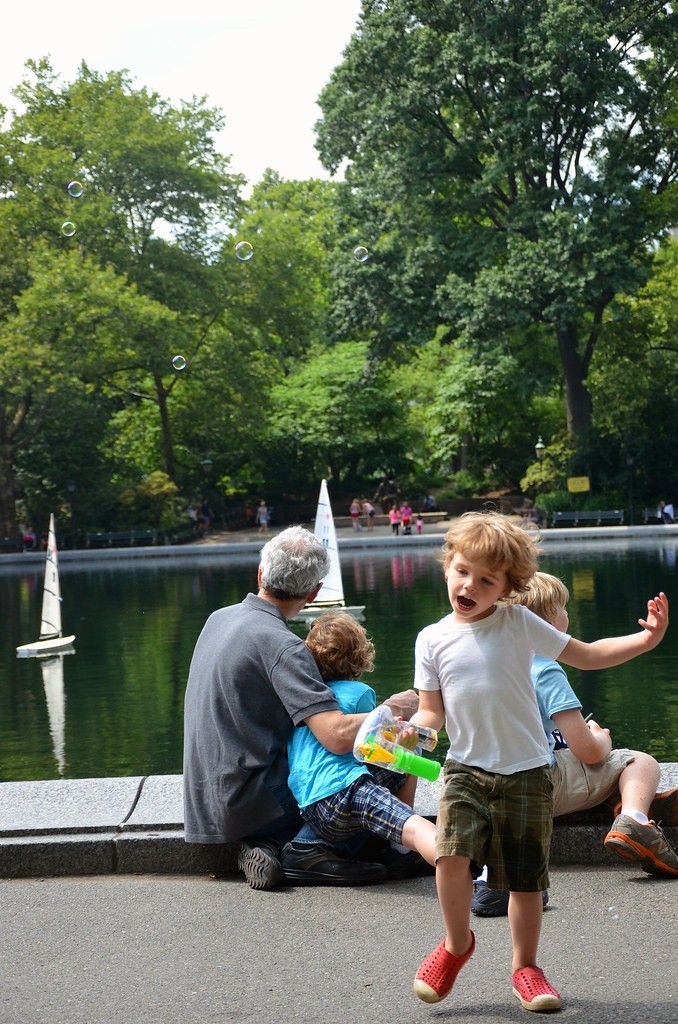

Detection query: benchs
(642, 508), (678, 524)
(552, 511), (624, 526)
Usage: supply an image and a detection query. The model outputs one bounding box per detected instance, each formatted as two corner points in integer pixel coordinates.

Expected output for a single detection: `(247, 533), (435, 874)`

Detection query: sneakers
(603, 788), (678, 879)
(512, 966), (563, 1010)
(237, 840), (280, 889)
(279, 843), (390, 886)
(414, 929), (475, 1004)
(471, 880), (550, 917)
(382, 848), (435, 879)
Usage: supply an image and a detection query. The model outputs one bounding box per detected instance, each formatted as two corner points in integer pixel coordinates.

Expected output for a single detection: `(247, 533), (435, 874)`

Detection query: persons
(657, 497), (674, 524)
(256, 500), (272, 538)
(182, 527), (395, 891)
(197, 498), (214, 525)
(389, 508), (670, 1013)
(500, 570), (678, 881)
(287, 609), (548, 918)
(349, 493), (436, 536)
